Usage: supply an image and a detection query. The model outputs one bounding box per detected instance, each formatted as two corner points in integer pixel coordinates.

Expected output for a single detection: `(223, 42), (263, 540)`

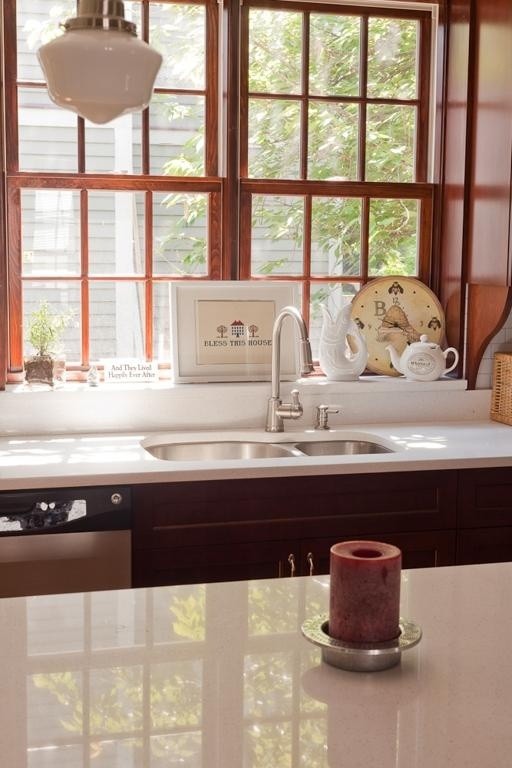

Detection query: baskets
(490, 351), (512, 426)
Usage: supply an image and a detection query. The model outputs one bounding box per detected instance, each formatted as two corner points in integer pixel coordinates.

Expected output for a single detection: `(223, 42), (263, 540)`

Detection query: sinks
(296, 437), (394, 455)
(147, 440), (293, 464)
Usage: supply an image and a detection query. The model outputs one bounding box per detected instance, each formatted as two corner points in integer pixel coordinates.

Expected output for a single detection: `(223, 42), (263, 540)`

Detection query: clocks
(346, 275), (444, 378)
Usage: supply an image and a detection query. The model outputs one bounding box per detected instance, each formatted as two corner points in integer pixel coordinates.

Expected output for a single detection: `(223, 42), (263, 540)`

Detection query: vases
(317, 298), (368, 382)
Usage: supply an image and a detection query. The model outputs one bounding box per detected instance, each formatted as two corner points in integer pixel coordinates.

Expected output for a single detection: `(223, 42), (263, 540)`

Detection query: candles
(328, 540), (403, 645)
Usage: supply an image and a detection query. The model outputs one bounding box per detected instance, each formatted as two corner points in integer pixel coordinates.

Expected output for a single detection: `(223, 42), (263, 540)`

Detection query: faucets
(270, 308), (314, 397)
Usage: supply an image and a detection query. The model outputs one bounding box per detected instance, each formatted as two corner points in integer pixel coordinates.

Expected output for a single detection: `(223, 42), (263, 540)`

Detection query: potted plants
(22, 300), (69, 387)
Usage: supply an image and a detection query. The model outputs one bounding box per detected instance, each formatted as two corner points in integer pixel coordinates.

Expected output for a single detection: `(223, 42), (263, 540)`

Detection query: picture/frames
(170, 278), (305, 384)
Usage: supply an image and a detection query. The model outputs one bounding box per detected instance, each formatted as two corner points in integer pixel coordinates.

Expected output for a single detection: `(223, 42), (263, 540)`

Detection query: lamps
(35, 0), (163, 124)
